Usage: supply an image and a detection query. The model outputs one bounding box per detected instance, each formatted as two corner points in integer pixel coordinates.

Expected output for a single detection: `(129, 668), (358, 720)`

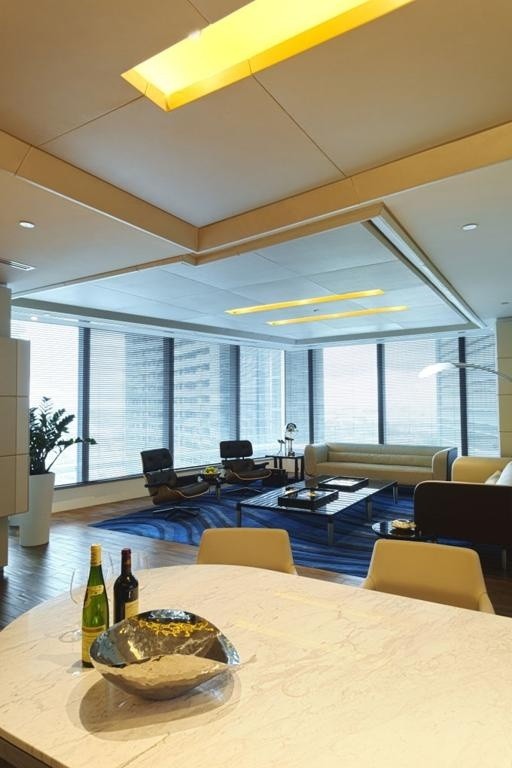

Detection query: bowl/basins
(90, 607), (240, 703)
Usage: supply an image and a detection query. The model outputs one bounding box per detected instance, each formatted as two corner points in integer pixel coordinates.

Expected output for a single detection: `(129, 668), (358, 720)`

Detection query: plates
(392, 525), (416, 530)
(203, 472), (221, 475)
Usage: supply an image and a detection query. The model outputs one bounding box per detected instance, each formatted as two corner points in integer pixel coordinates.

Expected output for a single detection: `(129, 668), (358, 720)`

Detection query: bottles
(114, 550), (139, 621)
(80, 544), (109, 666)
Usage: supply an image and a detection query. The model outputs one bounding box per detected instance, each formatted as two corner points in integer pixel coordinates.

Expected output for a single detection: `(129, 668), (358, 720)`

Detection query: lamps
(417, 362), (512, 386)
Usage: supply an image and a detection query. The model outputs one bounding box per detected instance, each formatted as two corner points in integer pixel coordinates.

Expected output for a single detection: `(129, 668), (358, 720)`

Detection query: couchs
(304, 441), (458, 486)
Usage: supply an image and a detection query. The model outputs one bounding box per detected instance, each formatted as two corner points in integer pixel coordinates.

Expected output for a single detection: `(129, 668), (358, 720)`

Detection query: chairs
(356, 538), (496, 615)
(413, 455), (512, 570)
(196, 527), (299, 575)
(140, 448), (210, 505)
(220, 440), (273, 483)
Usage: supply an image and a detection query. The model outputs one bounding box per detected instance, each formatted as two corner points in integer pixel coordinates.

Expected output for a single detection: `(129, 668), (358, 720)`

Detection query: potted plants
(18, 396), (99, 547)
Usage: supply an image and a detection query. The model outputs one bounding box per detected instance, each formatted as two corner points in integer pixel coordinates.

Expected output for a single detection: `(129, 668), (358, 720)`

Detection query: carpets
(88, 475), (424, 578)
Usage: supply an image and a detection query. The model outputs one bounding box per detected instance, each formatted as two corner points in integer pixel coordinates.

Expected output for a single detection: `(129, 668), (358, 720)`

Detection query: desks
(198, 477), (227, 497)
(265, 454), (304, 481)
(372, 518), (439, 540)
